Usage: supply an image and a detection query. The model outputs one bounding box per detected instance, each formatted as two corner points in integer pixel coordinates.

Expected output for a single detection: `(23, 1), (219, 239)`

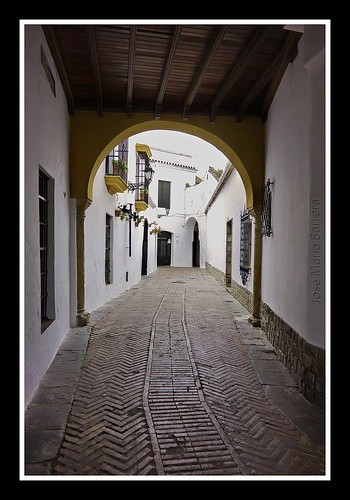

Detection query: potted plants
(112, 160), (128, 179)
